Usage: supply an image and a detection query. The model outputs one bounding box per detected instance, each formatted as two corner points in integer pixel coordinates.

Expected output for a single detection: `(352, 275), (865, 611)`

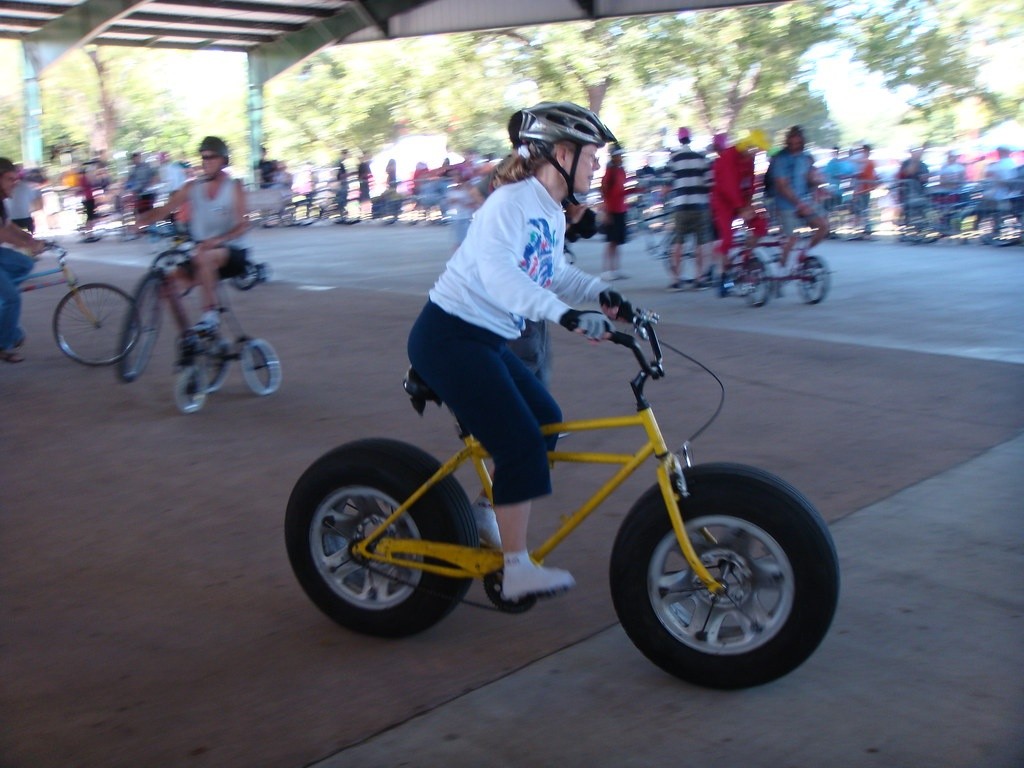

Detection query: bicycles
(10, 238), (142, 368)
(645, 194), (832, 307)
(262, 181), (363, 229)
(284, 304), (841, 691)
(585, 166), (1023, 248)
(116, 228), (282, 400)
(147, 214), (262, 302)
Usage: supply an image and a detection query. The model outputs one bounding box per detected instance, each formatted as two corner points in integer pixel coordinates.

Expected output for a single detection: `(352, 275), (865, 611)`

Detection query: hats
(678, 127), (689, 139)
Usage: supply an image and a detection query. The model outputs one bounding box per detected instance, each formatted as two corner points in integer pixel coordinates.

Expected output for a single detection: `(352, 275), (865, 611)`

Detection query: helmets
(714, 134), (737, 149)
(198, 137), (229, 167)
(509, 101), (618, 147)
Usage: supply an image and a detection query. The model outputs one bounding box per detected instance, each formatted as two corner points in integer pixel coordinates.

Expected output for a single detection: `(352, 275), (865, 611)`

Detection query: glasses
(581, 150), (600, 165)
(202, 153), (217, 159)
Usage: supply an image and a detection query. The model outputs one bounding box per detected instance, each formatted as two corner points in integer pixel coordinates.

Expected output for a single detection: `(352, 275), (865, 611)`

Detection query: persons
(0, 156), (49, 364)
(403, 98), (619, 603)
(126, 134), (252, 370)
(477, 109), (606, 439)
(0, 124), (1024, 300)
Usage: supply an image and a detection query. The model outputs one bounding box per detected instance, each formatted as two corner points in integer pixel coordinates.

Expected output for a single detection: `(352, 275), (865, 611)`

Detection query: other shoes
(2, 353), (24, 363)
(665, 283), (683, 291)
(601, 269), (631, 281)
(692, 281), (710, 291)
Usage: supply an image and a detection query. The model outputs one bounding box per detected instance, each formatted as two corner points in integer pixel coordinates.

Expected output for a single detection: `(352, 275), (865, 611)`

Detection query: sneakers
(472, 501), (502, 550)
(502, 567), (575, 596)
(191, 311), (220, 332)
